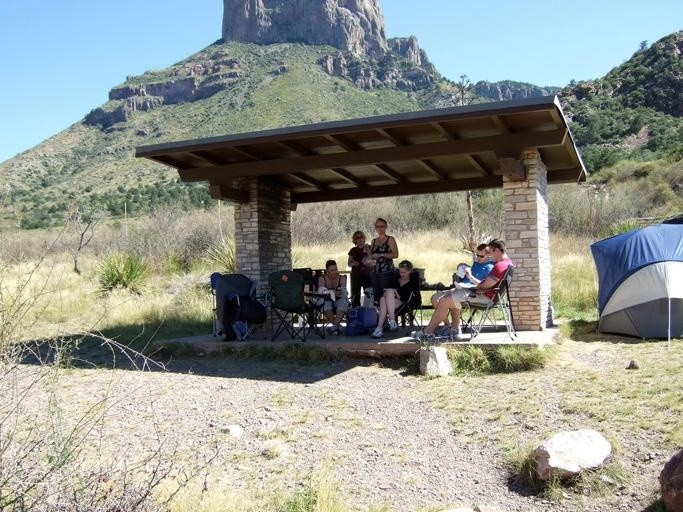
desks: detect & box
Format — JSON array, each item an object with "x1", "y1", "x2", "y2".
[{"x1": 395, "y1": 285, "x2": 453, "y2": 330}]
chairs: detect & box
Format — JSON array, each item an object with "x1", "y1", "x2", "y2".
[
  {"x1": 447, "y1": 264, "x2": 516, "y2": 343},
  {"x1": 210, "y1": 272, "x2": 270, "y2": 344},
  {"x1": 373, "y1": 269, "x2": 420, "y2": 336},
  {"x1": 268, "y1": 267, "x2": 348, "y2": 342}
]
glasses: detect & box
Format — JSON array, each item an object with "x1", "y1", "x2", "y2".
[
  {"x1": 399, "y1": 263, "x2": 410, "y2": 268},
  {"x1": 477, "y1": 254, "x2": 484, "y2": 258}
]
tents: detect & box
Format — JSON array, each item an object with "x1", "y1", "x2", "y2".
[{"x1": 586, "y1": 222, "x2": 683, "y2": 342}]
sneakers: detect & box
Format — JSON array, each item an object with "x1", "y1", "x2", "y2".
[
  {"x1": 389, "y1": 323, "x2": 399, "y2": 331},
  {"x1": 329, "y1": 325, "x2": 345, "y2": 336},
  {"x1": 411, "y1": 326, "x2": 462, "y2": 343},
  {"x1": 371, "y1": 328, "x2": 384, "y2": 338}
]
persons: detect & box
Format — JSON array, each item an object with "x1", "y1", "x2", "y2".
[
  {"x1": 370, "y1": 259, "x2": 421, "y2": 339},
  {"x1": 346, "y1": 229, "x2": 371, "y2": 306},
  {"x1": 362, "y1": 217, "x2": 398, "y2": 303},
  {"x1": 411, "y1": 238, "x2": 513, "y2": 340},
  {"x1": 430, "y1": 242, "x2": 493, "y2": 334},
  {"x1": 318, "y1": 259, "x2": 347, "y2": 335}
]
